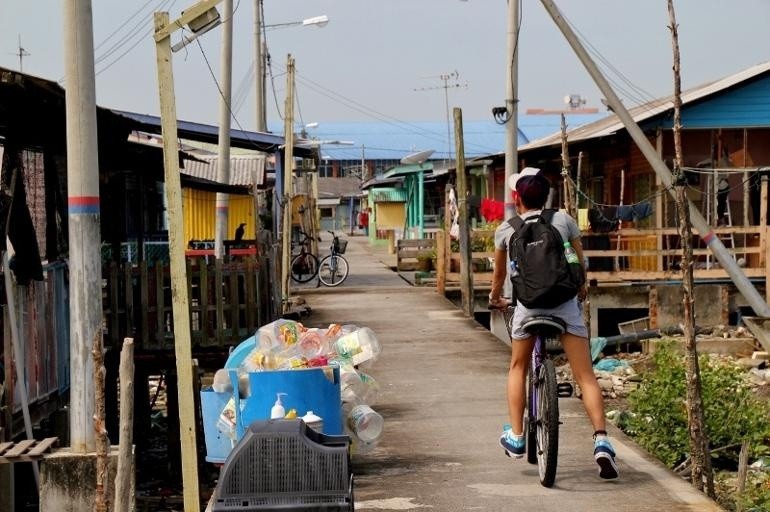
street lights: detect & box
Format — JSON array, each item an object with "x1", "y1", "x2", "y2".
[
  {"x1": 279, "y1": 140, "x2": 355, "y2": 312},
  {"x1": 254, "y1": 14, "x2": 331, "y2": 132},
  {"x1": 292, "y1": 122, "x2": 320, "y2": 137}
]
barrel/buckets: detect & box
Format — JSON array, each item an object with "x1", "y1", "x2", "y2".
[{"x1": 216, "y1": 318, "x2": 385, "y2": 443}]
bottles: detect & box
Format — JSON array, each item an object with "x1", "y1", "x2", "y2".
[
  {"x1": 285, "y1": 408, "x2": 297, "y2": 418},
  {"x1": 508, "y1": 261, "x2": 521, "y2": 278},
  {"x1": 563, "y1": 241, "x2": 580, "y2": 266},
  {"x1": 301, "y1": 411, "x2": 324, "y2": 433}
]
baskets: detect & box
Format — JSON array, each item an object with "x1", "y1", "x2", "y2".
[{"x1": 334, "y1": 239, "x2": 348, "y2": 254}]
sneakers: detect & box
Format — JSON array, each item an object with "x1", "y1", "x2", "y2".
[
  {"x1": 594, "y1": 440, "x2": 619, "y2": 481},
  {"x1": 499, "y1": 430, "x2": 526, "y2": 456}
]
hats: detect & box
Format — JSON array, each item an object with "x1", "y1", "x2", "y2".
[{"x1": 516, "y1": 175, "x2": 549, "y2": 196}]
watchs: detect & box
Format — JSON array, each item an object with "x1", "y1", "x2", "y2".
[{"x1": 488, "y1": 294, "x2": 501, "y2": 304}]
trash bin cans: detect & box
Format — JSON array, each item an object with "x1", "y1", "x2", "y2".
[{"x1": 210, "y1": 417, "x2": 354, "y2": 512}]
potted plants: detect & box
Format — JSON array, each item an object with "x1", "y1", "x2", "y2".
[{"x1": 416, "y1": 233, "x2": 487, "y2": 272}]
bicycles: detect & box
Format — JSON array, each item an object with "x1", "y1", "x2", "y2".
[
  {"x1": 290, "y1": 231, "x2": 320, "y2": 284},
  {"x1": 487, "y1": 291, "x2": 587, "y2": 487},
  {"x1": 318, "y1": 230, "x2": 350, "y2": 288}
]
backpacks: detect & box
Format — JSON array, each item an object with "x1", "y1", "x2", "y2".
[{"x1": 507, "y1": 209, "x2": 585, "y2": 309}]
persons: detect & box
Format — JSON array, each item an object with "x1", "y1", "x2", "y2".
[{"x1": 488, "y1": 174, "x2": 619, "y2": 479}]
